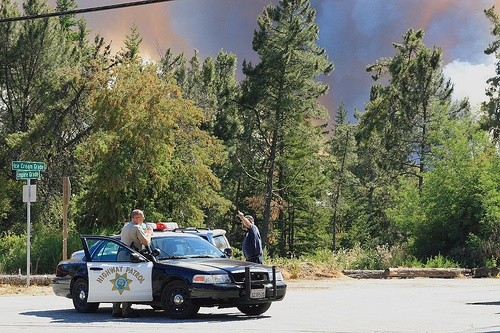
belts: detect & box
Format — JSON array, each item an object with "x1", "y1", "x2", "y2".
[{"x1": 246, "y1": 255, "x2": 260, "y2": 261}]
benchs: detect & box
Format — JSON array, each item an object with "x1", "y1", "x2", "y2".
[{"x1": 98, "y1": 254, "x2": 117, "y2": 261}]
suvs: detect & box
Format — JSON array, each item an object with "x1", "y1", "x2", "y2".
[{"x1": 70, "y1": 223, "x2": 233, "y2": 260}]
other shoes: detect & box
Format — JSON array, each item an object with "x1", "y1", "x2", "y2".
[
  {"x1": 112, "y1": 312, "x2": 123, "y2": 318},
  {"x1": 122, "y1": 310, "x2": 140, "y2": 318}
]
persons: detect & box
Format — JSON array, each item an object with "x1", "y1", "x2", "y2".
[
  {"x1": 236, "y1": 210, "x2": 263, "y2": 264},
  {"x1": 112, "y1": 209, "x2": 153, "y2": 318}
]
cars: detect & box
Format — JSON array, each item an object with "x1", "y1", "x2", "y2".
[{"x1": 51, "y1": 232, "x2": 287, "y2": 320}]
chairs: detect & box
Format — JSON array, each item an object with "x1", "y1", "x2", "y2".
[{"x1": 160, "y1": 239, "x2": 177, "y2": 257}]
